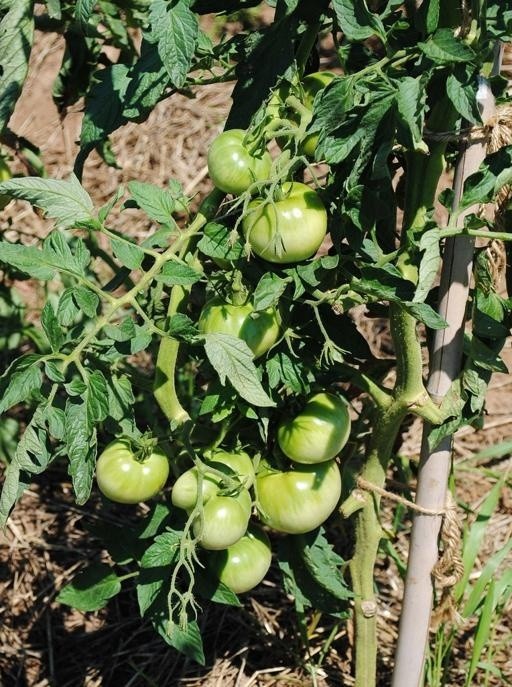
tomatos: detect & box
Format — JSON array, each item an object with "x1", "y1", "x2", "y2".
[{"x1": 95, "y1": 72, "x2": 352, "y2": 593}]
